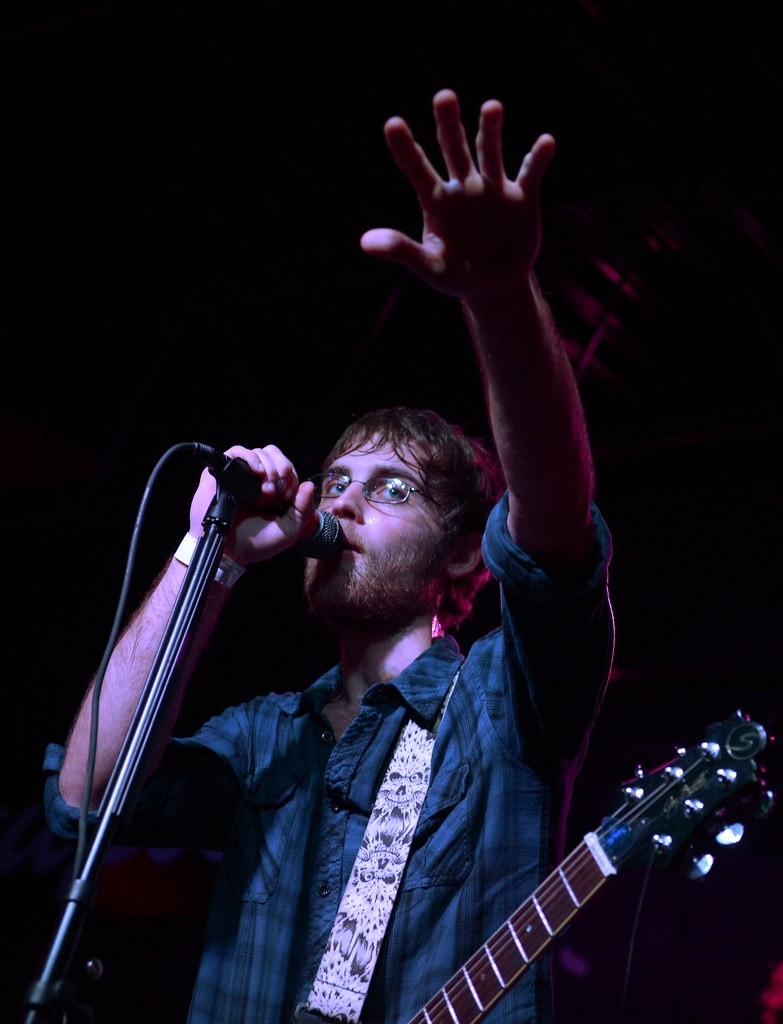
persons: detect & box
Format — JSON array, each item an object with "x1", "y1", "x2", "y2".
[{"x1": 41, "y1": 88, "x2": 614, "y2": 1024}]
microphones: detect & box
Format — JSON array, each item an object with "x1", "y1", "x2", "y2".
[{"x1": 191, "y1": 442, "x2": 343, "y2": 558}]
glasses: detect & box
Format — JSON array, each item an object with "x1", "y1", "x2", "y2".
[{"x1": 306, "y1": 472, "x2": 449, "y2": 515}]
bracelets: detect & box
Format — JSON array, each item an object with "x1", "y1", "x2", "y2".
[{"x1": 174, "y1": 532, "x2": 247, "y2": 587}]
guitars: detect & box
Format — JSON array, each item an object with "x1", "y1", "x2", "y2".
[{"x1": 400, "y1": 707, "x2": 773, "y2": 1022}]
